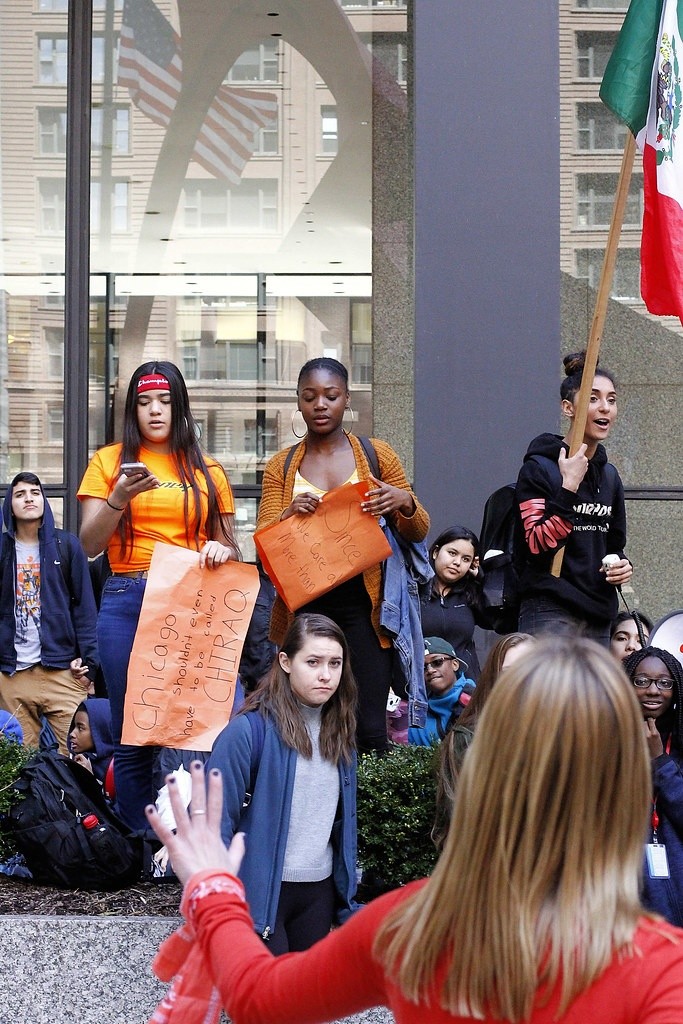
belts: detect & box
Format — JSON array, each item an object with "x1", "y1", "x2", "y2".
[{"x1": 112, "y1": 572, "x2": 149, "y2": 579}]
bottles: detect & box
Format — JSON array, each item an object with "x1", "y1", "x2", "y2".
[{"x1": 83, "y1": 815, "x2": 132, "y2": 875}]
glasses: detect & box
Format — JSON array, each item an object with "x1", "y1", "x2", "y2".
[
  {"x1": 632, "y1": 675, "x2": 676, "y2": 690},
  {"x1": 424, "y1": 657, "x2": 455, "y2": 672}
]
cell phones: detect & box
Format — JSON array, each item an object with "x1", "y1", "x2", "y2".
[{"x1": 121, "y1": 463, "x2": 160, "y2": 485}]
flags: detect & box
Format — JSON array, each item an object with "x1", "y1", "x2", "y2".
[{"x1": 599, "y1": 0, "x2": 683, "y2": 328}]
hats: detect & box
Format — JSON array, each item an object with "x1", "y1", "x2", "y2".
[{"x1": 421, "y1": 637, "x2": 469, "y2": 673}]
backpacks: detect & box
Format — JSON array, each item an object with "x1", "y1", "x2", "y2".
[
  {"x1": 9, "y1": 753, "x2": 144, "y2": 889},
  {"x1": 472, "y1": 455, "x2": 616, "y2": 636}
]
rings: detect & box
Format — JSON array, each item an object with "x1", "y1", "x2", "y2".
[{"x1": 191, "y1": 810, "x2": 206, "y2": 816}]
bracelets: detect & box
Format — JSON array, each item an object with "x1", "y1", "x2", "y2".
[{"x1": 107, "y1": 500, "x2": 125, "y2": 511}]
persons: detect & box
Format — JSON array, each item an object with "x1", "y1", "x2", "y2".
[
  {"x1": 610, "y1": 609, "x2": 654, "y2": 662},
  {"x1": 237, "y1": 557, "x2": 278, "y2": 699},
  {"x1": 408, "y1": 638, "x2": 476, "y2": 748},
  {"x1": 417, "y1": 525, "x2": 495, "y2": 687},
  {"x1": 255, "y1": 357, "x2": 430, "y2": 760},
  {"x1": 145, "y1": 638, "x2": 683, "y2": 1024},
  {"x1": 203, "y1": 614, "x2": 368, "y2": 958},
  {"x1": 621, "y1": 646, "x2": 683, "y2": 929},
  {"x1": 0, "y1": 472, "x2": 113, "y2": 764},
  {"x1": 66, "y1": 698, "x2": 116, "y2": 805},
  {"x1": 512, "y1": 350, "x2": 634, "y2": 650},
  {"x1": 76, "y1": 361, "x2": 243, "y2": 830}
]
